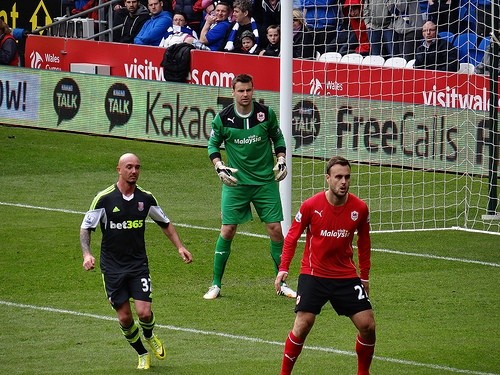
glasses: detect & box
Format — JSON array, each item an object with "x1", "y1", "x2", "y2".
[{"x1": 173, "y1": 19, "x2": 185, "y2": 22}]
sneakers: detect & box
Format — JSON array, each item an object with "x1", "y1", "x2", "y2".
[
  {"x1": 143, "y1": 333, "x2": 166, "y2": 359},
  {"x1": 277, "y1": 284, "x2": 297, "y2": 298},
  {"x1": 203, "y1": 285, "x2": 221, "y2": 300},
  {"x1": 137, "y1": 353, "x2": 150, "y2": 370}
]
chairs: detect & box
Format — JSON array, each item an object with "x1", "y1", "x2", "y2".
[{"x1": 188, "y1": 1, "x2": 495, "y2": 71}]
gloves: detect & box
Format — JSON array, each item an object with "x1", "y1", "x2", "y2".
[
  {"x1": 215, "y1": 160, "x2": 238, "y2": 188},
  {"x1": 272, "y1": 156, "x2": 287, "y2": 182}
]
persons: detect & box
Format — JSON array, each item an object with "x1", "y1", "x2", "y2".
[
  {"x1": 199, "y1": 74, "x2": 299, "y2": 299},
  {"x1": 78, "y1": 151, "x2": 195, "y2": 371},
  {"x1": 0, "y1": 19, "x2": 20, "y2": 66},
  {"x1": 68, "y1": 0, "x2": 499, "y2": 78},
  {"x1": 273, "y1": 157, "x2": 380, "y2": 375}
]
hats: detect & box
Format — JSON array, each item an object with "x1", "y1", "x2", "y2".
[{"x1": 241, "y1": 30, "x2": 255, "y2": 41}]
